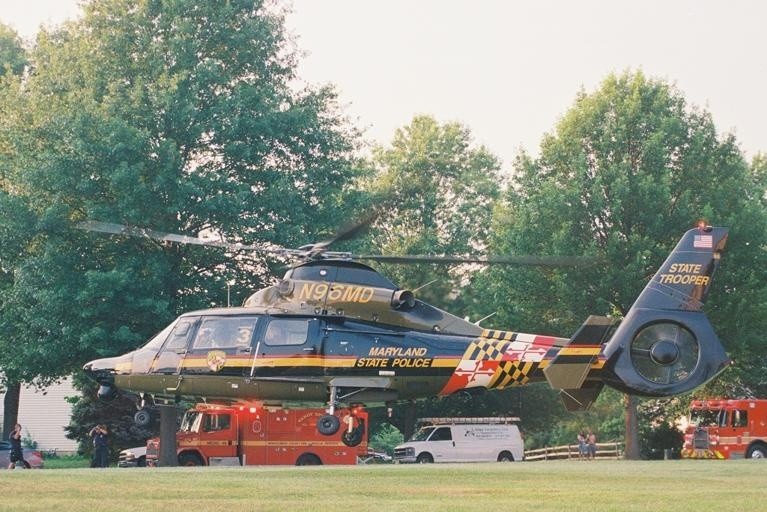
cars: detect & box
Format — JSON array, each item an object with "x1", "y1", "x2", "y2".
[
  {"x1": 0, "y1": 439, "x2": 45, "y2": 469},
  {"x1": 117, "y1": 446, "x2": 149, "y2": 468}
]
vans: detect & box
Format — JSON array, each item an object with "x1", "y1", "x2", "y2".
[{"x1": 391, "y1": 421, "x2": 526, "y2": 464}]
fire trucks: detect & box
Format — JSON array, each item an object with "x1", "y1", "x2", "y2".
[
  {"x1": 681, "y1": 398, "x2": 767, "y2": 459},
  {"x1": 143, "y1": 402, "x2": 368, "y2": 468}
]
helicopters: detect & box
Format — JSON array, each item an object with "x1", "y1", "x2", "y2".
[{"x1": 72, "y1": 206, "x2": 732, "y2": 446}]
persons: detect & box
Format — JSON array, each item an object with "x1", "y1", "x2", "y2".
[
  {"x1": 7, "y1": 424, "x2": 32, "y2": 470},
  {"x1": 577, "y1": 429, "x2": 598, "y2": 460},
  {"x1": 89, "y1": 424, "x2": 112, "y2": 468}
]
